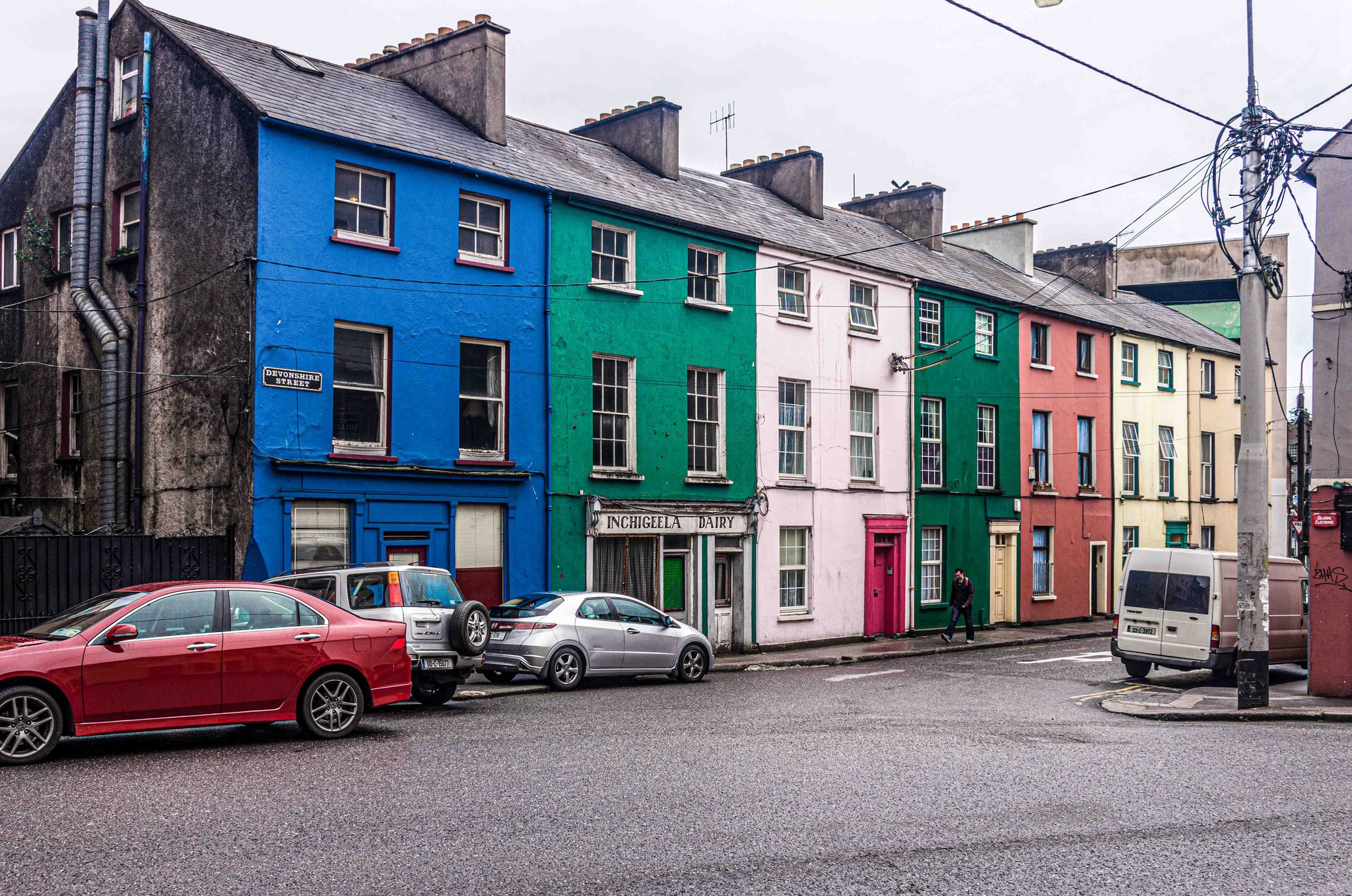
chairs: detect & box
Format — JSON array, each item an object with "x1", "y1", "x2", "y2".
[
  {"x1": 594, "y1": 602, "x2": 611, "y2": 620},
  {"x1": 173, "y1": 611, "x2": 205, "y2": 635},
  {"x1": 301, "y1": 588, "x2": 374, "y2": 608}
]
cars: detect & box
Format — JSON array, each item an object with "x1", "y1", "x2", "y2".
[
  {"x1": 0, "y1": 578, "x2": 414, "y2": 765},
  {"x1": 474, "y1": 590, "x2": 715, "y2": 691}
]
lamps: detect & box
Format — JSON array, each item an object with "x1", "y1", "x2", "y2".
[{"x1": 462, "y1": 400, "x2": 486, "y2": 450}]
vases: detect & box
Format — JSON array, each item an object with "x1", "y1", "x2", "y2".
[
  {"x1": 1079, "y1": 488, "x2": 1095, "y2": 494},
  {"x1": 1033, "y1": 485, "x2": 1052, "y2": 492},
  {"x1": 5, "y1": 473, "x2": 18, "y2": 481}
]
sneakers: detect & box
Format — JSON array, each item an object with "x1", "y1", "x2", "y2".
[
  {"x1": 966, "y1": 639, "x2": 975, "y2": 643},
  {"x1": 941, "y1": 633, "x2": 951, "y2": 643}
]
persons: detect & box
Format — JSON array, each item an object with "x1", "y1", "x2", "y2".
[{"x1": 941, "y1": 568, "x2": 975, "y2": 644}]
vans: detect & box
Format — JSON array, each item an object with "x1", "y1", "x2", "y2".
[{"x1": 1110, "y1": 546, "x2": 1309, "y2": 687}]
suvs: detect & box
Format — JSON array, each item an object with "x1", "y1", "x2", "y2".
[{"x1": 229, "y1": 561, "x2": 492, "y2": 704}]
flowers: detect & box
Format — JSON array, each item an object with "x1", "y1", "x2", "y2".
[
  {"x1": 7, "y1": 454, "x2": 17, "y2": 474},
  {"x1": 1033, "y1": 481, "x2": 1053, "y2": 487},
  {"x1": 1078, "y1": 483, "x2": 1096, "y2": 489}
]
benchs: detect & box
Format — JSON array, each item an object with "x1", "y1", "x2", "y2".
[{"x1": 248, "y1": 608, "x2": 312, "y2": 630}]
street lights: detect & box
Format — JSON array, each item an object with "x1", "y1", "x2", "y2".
[{"x1": 1032, "y1": 0, "x2": 1270, "y2": 709}]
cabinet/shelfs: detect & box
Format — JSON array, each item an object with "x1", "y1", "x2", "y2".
[{"x1": 334, "y1": 412, "x2": 362, "y2": 440}]
